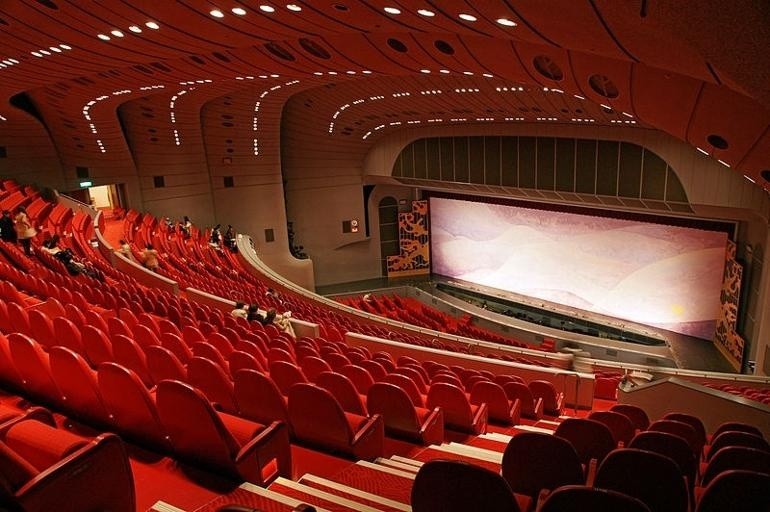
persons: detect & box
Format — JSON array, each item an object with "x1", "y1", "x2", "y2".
[
  {"x1": 363, "y1": 292, "x2": 373, "y2": 305},
  {"x1": 0, "y1": 206, "x2": 86, "y2": 276},
  {"x1": 116, "y1": 239, "x2": 142, "y2": 266},
  {"x1": 142, "y1": 243, "x2": 159, "y2": 272},
  {"x1": 266, "y1": 288, "x2": 279, "y2": 297},
  {"x1": 230, "y1": 300, "x2": 295, "y2": 341},
  {"x1": 165, "y1": 216, "x2": 238, "y2": 256}
]
